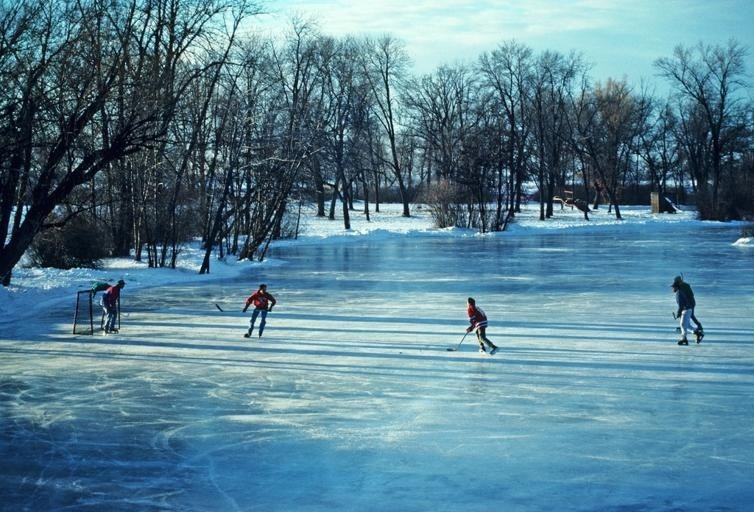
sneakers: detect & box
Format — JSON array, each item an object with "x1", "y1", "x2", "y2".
[{"x1": 104, "y1": 327, "x2": 119, "y2": 331}]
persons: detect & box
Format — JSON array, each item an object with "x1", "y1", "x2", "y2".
[
  {"x1": 100, "y1": 280, "x2": 125, "y2": 334},
  {"x1": 242, "y1": 284, "x2": 276, "y2": 338},
  {"x1": 466, "y1": 298, "x2": 497, "y2": 354},
  {"x1": 669, "y1": 281, "x2": 702, "y2": 343},
  {"x1": 675, "y1": 277, "x2": 703, "y2": 330}
]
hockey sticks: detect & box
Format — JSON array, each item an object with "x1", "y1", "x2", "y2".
[
  {"x1": 216, "y1": 304, "x2": 270, "y2": 312},
  {"x1": 672, "y1": 312, "x2": 680, "y2": 320},
  {"x1": 447, "y1": 332, "x2": 470, "y2": 351}
]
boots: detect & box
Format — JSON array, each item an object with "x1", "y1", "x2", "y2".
[
  {"x1": 479, "y1": 347, "x2": 499, "y2": 355},
  {"x1": 694, "y1": 327, "x2": 704, "y2": 343},
  {"x1": 678, "y1": 340, "x2": 688, "y2": 345}
]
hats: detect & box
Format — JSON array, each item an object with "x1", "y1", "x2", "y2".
[
  {"x1": 117, "y1": 280, "x2": 126, "y2": 284},
  {"x1": 669, "y1": 282, "x2": 679, "y2": 287}
]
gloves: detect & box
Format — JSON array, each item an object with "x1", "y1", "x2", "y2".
[{"x1": 677, "y1": 310, "x2": 680, "y2": 317}]
